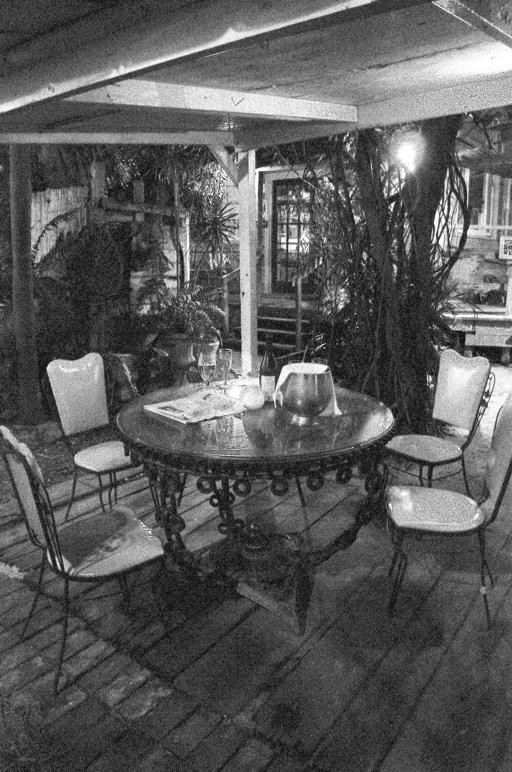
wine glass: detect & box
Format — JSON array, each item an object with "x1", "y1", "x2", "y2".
[
  {"x1": 197, "y1": 353, "x2": 217, "y2": 401},
  {"x1": 217, "y1": 348, "x2": 233, "y2": 389},
  {"x1": 280, "y1": 365, "x2": 334, "y2": 427}
]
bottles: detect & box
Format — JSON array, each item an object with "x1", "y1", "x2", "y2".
[{"x1": 258, "y1": 334, "x2": 277, "y2": 405}]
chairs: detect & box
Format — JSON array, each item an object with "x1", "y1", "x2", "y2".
[
  {"x1": 379, "y1": 347, "x2": 512, "y2": 633},
  {"x1": 45, "y1": 352, "x2": 148, "y2": 522},
  {"x1": 0, "y1": 425, "x2": 174, "y2": 698}
]
